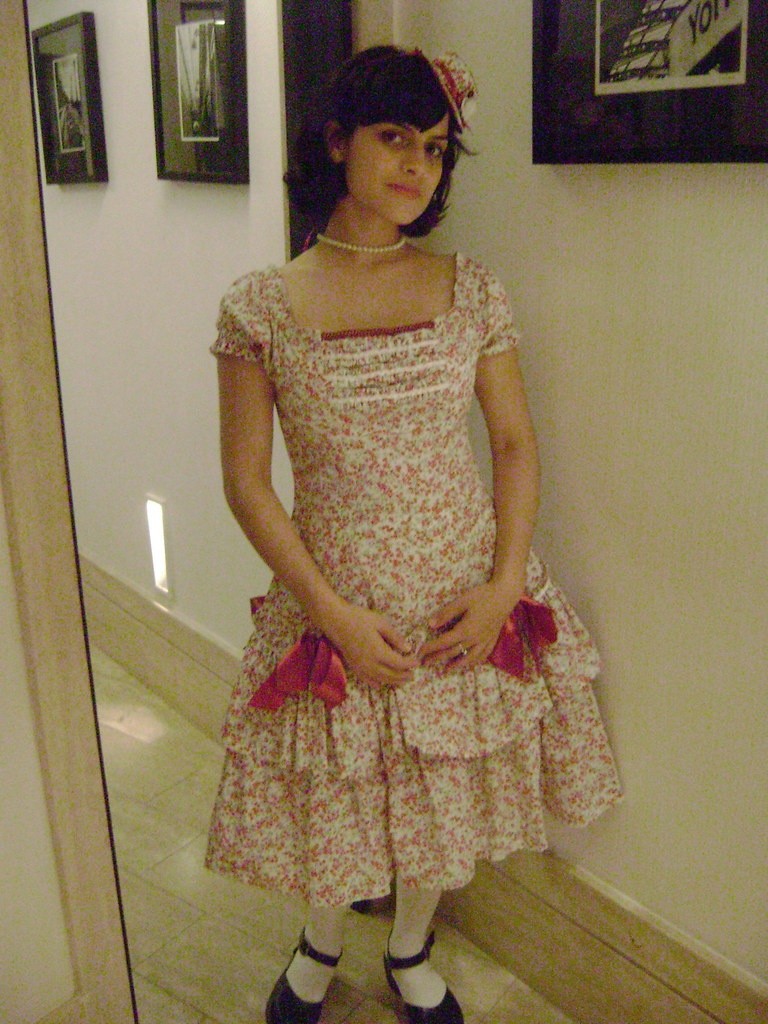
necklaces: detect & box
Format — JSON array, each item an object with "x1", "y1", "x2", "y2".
[{"x1": 318, "y1": 234, "x2": 407, "y2": 253}]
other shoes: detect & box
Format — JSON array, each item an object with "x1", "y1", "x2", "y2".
[
  {"x1": 382, "y1": 927, "x2": 465, "y2": 1024},
  {"x1": 265, "y1": 924, "x2": 344, "y2": 1024}
]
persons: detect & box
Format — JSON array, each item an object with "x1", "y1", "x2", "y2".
[{"x1": 205, "y1": 45, "x2": 625, "y2": 1024}]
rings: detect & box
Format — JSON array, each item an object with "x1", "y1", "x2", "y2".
[{"x1": 458, "y1": 643, "x2": 467, "y2": 656}]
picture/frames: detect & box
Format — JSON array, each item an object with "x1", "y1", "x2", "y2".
[
  {"x1": 532, "y1": 0, "x2": 768, "y2": 164},
  {"x1": 148, "y1": 0, "x2": 250, "y2": 186},
  {"x1": 32, "y1": 11, "x2": 107, "y2": 185}
]
